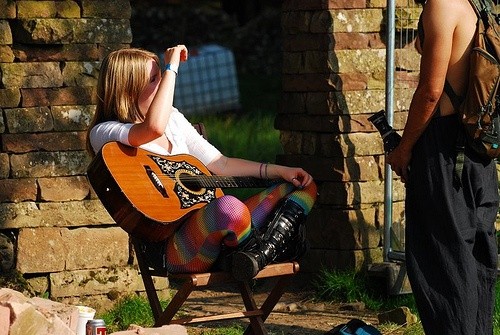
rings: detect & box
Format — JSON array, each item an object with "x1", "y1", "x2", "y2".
[{"x1": 291, "y1": 178, "x2": 296, "y2": 182}]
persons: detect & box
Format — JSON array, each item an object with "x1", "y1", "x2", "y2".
[
  {"x1": 386, "y1": 0, "x2": 500, "y2": 335},
  {"x1": 87, "y1": 45, "x2": 317, "y2": 279}
]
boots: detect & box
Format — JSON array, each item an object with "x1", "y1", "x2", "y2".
[{"x1": 229, "y1": 197, "x2": 306, "y2": 279}]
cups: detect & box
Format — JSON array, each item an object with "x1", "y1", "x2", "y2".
[{"x1": 74, "y1": 305, "x2": 96, "y2": 334}]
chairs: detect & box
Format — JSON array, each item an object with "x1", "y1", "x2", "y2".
[{"x1": 129, "y1": 236, "x2": 300, "y2": 335}]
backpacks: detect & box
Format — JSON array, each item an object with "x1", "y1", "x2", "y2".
[{"x1": 417, "y1": 0, "x2": 500, "y2": 159}]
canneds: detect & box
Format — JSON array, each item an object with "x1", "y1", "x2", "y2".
[{"x1": 86, "y1": 319, "x2": 107, "y2": 335}]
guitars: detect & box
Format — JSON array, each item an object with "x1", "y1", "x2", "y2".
[{"x1": 87, "y1": 141, "x2": 285, "y2": 246}]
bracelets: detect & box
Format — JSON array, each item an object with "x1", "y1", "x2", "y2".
[
  {"x1": 265, "y1": 163, "x2": 268, "y2": 180},
  {"x1": 260, "y1": 163, "x2": 263, "y2": 179},
  {"x1": 162, "y1": 64, "x2": 178, "y2": 76}
]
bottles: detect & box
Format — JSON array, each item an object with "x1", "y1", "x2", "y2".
[{"x1": 367, "y1": 109, "x2": 422, "y2": 183}]
